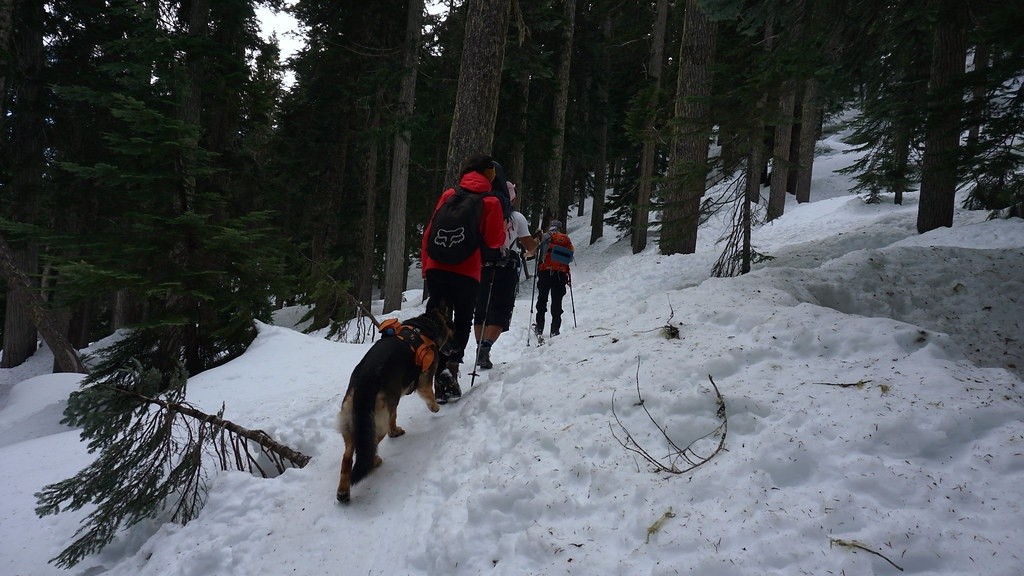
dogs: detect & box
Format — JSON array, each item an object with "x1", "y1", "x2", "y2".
[{"x1": 336, "y1": 308, "x2": 455, "y2": 503}]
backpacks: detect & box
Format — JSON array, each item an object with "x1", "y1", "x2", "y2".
[
  {"x1": 427, "y1": 186, "x2": 495, "y2": 264},
  {"x1": 545, "y1": 231, "x2": 574, "y2": 271}
]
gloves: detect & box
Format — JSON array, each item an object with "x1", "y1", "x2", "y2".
[{"x1": 534, "y1": 231, "x2": 543, "y2": 243}]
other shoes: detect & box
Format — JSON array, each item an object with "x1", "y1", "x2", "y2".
[
  {"x1": 549, "y1": 330, "x2": 560, "y2": 337},
  {"x1": 434, "y1": 381, "x2": 448, "y2": 405},
  {"x1": 437, "y1": 373, "x2": 462, "y2": 397},
  {"x1": 535, "y1": 328, "x2": 543, "y2": 336}
]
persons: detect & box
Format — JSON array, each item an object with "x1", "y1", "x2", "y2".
[
  {"x1": 421, "y1": 155, "x2": 507, "y2": 406},
  {"x1": 525, "y1": 219, "x2": 574, "y2": 338},
  {"x1": 474, "y1": 180, "x2": 543, "y2": 370}
]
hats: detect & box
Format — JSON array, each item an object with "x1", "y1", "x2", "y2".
[
  {"x1": 549, "y1": 220, "x2": 562, "y2": 228},
  {"x1": 505, "y1": 181, "x2": 516, "y2": 201}
]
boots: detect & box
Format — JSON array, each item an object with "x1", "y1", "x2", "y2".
[{"x1": 476, "y1": 342, "x2": 493, "y2": 369}]
